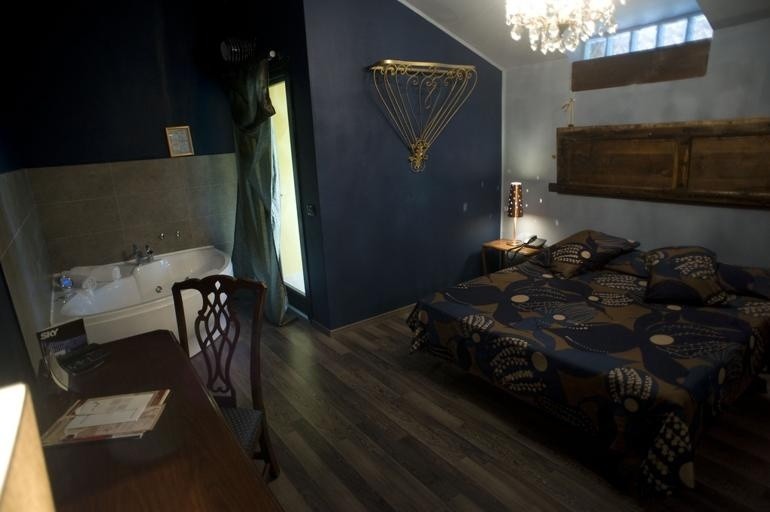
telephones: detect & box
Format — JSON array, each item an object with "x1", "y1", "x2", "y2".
[
  {"x1": 61, "y1": 343, "x2": 111, "y2": 372},
  {"x1": 524, "y1": 234, "x2": 547, "y2": 248}
]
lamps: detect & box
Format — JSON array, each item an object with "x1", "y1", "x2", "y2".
[
  {"x1": 507, "y1": 178, "x2": 522, "y2": 247},
  {"x1": 503, "y1": 0, "x2": 626, "y2": 56}
]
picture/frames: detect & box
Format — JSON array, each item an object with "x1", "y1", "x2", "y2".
[{"x1": 164, "y1": 125, "x2": 195, "y2": 158}]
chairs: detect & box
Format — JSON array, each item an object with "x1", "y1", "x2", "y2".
[{"x1": 170, "y1": 274, "x2": 280, "y2": 486}]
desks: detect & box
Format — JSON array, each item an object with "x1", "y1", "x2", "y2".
[
  {"x1": 483, "y1": 237, "x2": 542, "y2": 272},
  {"x1": 30, "y1": 326, "x2": 281, "y2": 512}
]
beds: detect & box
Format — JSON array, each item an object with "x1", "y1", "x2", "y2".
[{"x1": 405, "y1": 245, "x2": 770, "y2": 512}]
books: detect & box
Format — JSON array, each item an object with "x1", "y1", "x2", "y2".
[
  {"x1": 36, "y1": 316, "x2": 89, "y2": 372},
  {"x1": 42, "y1": 344, "x2": 174, "y2": 449}
]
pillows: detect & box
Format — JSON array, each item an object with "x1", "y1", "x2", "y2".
[
  {"x1": 526, "y1": 230, "x2": 641, "y2": 282},
  {"x1": 641, "y1": 244, "x2": 736, "y2": 305}
]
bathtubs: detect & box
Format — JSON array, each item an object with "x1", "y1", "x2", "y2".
[{"x1": 50, "y1": 245, "x2": 234, "y2": 358}]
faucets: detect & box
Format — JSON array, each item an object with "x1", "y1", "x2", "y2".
[
  {"x1": 145, "y1": 245, "x2": 154, "y2": 262},
  {"x1": 129, "y1": 243, "x2": 143, "y2": 264}
]
